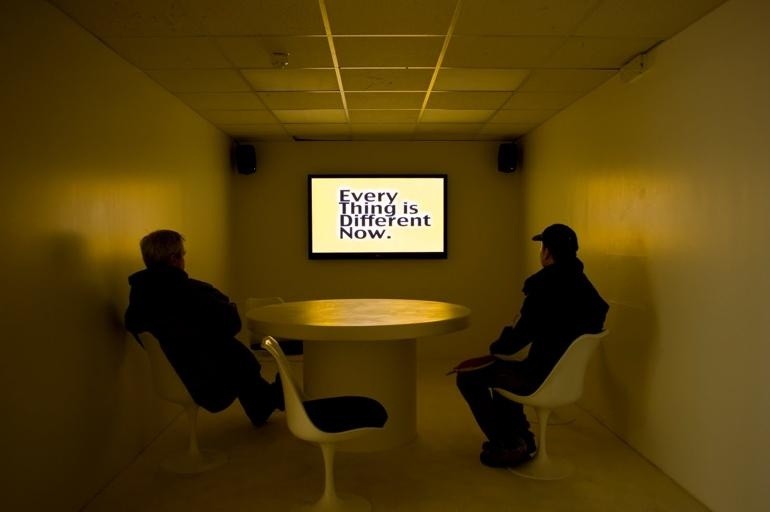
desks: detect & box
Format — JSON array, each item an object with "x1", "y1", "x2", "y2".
[{"x1": 247, "y1": 298, "x2": 471, "y2": 451}]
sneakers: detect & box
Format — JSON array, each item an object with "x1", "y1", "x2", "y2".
[{"x1": 480, "y1": 448, "x2": 525, "y2": 467}]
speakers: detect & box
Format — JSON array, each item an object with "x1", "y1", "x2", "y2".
[
  {"x1": 235, "y1": 144, "x2": 256, "y2": 175},
  {"x1": 497, "y1": 143, "x2": 519, "y2": 173}
]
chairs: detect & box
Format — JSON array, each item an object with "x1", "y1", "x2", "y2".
[
  {"x1": 496, "y1": 329, "x2": 611, "y2": 481},
  {"x1": 117, "y1": 332, "x2": 213, "y2": 479},
  {"x1": 248, "y1": 332, "x2": 303, "y2": 357},
  {"x1": 257, "y1": 337, "x2": 389, "y2": 507}
]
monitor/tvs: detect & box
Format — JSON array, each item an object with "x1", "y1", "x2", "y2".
[{"x1": 308, "y1": 173, "x2": 448, "y2": 260}]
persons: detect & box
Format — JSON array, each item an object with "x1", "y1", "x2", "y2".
[
  {"x1": 125, "y1": 229, "x2": 285, "y2": 428},
  {"x1": 456, "y1": 223, "x2": 609, "y2": 468}
]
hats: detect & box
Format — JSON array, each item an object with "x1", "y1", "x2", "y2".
[{"x1": 532, "y1": 223, "x2": 579, "y2": 250}]
